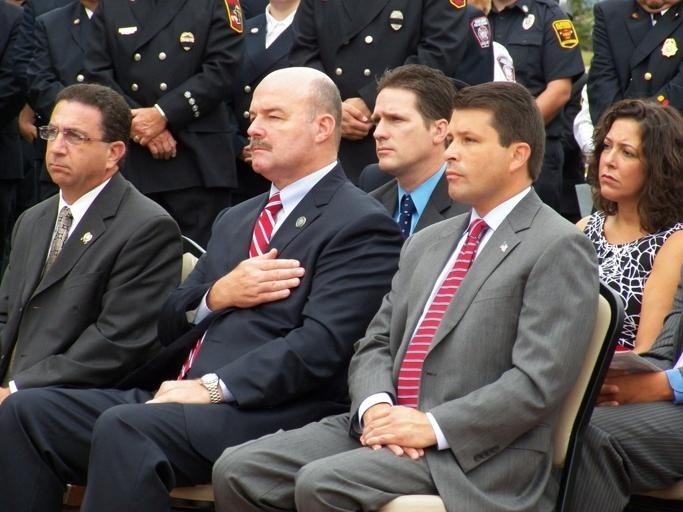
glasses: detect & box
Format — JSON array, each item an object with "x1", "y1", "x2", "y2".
[{"x1": 38, "y1": 124, "x2": 110, "y2": 147}]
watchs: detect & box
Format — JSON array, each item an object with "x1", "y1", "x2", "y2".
[{"x1": 198, "y1": 372, "x2": 219, "y2": 405}]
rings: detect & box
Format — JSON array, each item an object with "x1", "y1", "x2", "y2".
[{"x1": 135, "y1": 136, "x2": 139, "y2": 140}]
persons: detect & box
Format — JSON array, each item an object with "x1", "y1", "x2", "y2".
[
  {"x1": 572, "y1": 97, "x2": 682, "y2": 354},
  {"x1": 2, "y1": 4, "x2": 586, "y2": 273},
  {"x1": 562, "y1": 263, "x2": 683, "y2": 512},
  {"x1": 1, "y1": 66, "x2": 400, "y2": 511},
  {"x1": 587, "y1": 3, "x2": 680, "y2": 126},
  {"x1": 358, "y1": 63, "x2": 469, "y2": 238},
  {"x1": 0, "y1": 83, "x2": 183, "y2": 400},
  {"x1": 213, "y1": 79, "x2": 601, "y2": 512}
]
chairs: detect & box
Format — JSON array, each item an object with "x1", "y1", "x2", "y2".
[
  {"x1": 378, "y1": 276, "x2": 626, "y2": 511},
  {"x1": 174, "y1": 235, "x2": 210, "y2": 286}
]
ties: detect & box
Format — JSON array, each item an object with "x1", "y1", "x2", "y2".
[
  {"x1": 42, "y1": 205, "x2": 74, "y2": 283},
  {"x1": 398, "y1": 193, "x2": 418, "y2": 242},
  {"x1": 176, "y1": 190, "x2": 284, "y2": 383},
  {"x1": 395, "y1": 217, "x2": 492, "y2": 413}
]
